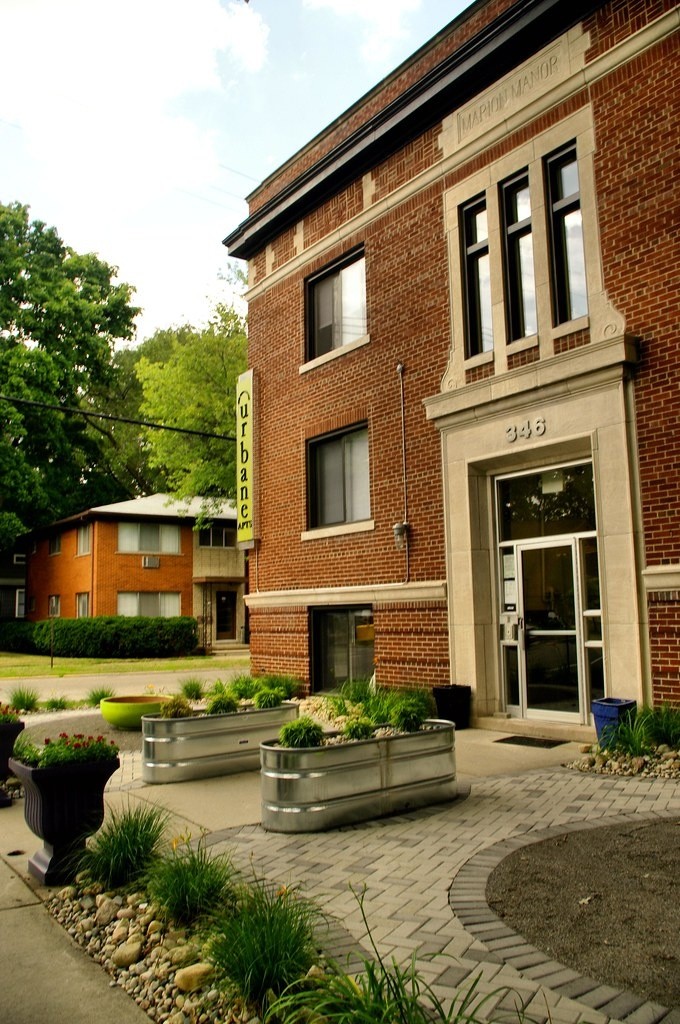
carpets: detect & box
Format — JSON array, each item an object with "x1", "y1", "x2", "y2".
[{"x1": 494, "y1": 736, "x2": 570, "y2": 749}]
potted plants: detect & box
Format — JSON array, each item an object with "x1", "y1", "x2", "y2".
[
  {"x1": 259, "y1": 700, "x2": 456, "y2": 832},
  {"x1": 141, "y1": 691, "x2": 300, "y2": 784}
]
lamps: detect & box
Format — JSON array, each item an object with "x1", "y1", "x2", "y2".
[{"x1": 392, "y1": 522, "x2": 405, "y2": 549}]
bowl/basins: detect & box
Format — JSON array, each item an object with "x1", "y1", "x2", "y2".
[{"x1": 100, "y1": 694, "x2": 175, "y2": 731}]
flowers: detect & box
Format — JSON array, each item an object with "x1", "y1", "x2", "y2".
[
  {"x1": 0, "y1": 702, "x2": 19, "y2": 724},
  {"x1": 24, "y1": 732, "x2": 118, "y2": 767}
]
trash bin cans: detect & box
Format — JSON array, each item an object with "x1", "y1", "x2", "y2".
[
  {"x1": 591, "y1": 697, "x2": 637, "y2": 751},
  {"x1": 433, "y1": 684, "x2": 471, "y2": 730}
]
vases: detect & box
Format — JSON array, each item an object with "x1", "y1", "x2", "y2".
[
  {"x1": 100, "y1": 695, "x2": 173, "y2": 731},
  {"x1": 9, "y1": 758, "x2": 120, "y2": 885},
  {"x1": 592, "y1": 698, "x2": 636, "y2": 750},
  {"x1": 433, "y1": 685, "x2": 471, "y2": 729},
  {"x1": 0, "y1": 723, "x2": 24, "y2": 807}
]
